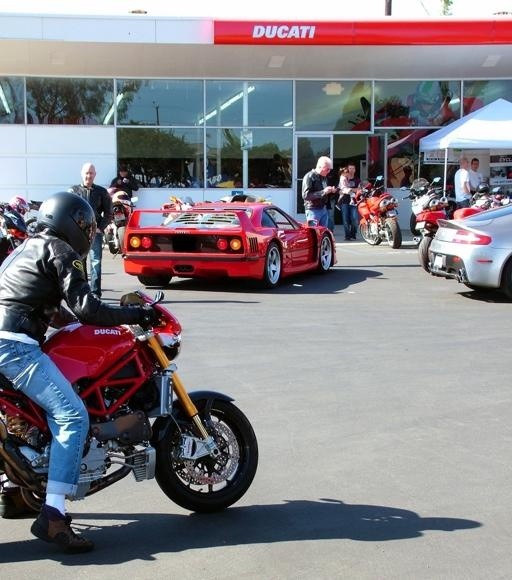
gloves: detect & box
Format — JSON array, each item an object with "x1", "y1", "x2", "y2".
[{"x1": 139, "y1": 305, "x2": 162, "y2": 329}]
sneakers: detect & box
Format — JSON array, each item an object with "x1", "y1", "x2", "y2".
[
  {"x1": 335, "y1": 204, "x2": 341, "y2": 211},
  {"x1": 349, "y1": 202, "x2": 358, "y2": 207},
  {"x1": 31, "y1": 503, "x2": 94, "y2": 554},
  {"x1": 344, "y1": 236, "x2": 356, "y2": 241}
]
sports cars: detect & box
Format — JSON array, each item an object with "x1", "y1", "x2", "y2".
[{"x1": 112, "y1": 197, "x2": 341, "y2": 284}]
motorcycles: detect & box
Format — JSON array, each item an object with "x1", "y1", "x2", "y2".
[
  {"x1": 1, "y1": 288, "x2": 259, "y2": 521},
  {"x1": 0, "y1": 196, "x2": 139, "y2": 278},
  {"x1": 350, "y1": 176, "x2": 512, "y2": 272}
]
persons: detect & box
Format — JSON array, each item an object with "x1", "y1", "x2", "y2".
[
  {"x1": 392, "y1": 80, "x2": 458, "y2": 135},
  {"x1": 301, "y1": 156, "x2": 339, "y2": 232},
  {"x1": 468, "y1": 158, "x2": 483, "y2": 194},
  {"x1": 335, "y1": 166, "x2": 357, "y2": 210},
  {"x1": 108, "y1": 164, "x2": 139, "y2": 197},
  {"x1": 341, "y1": 163, "x2": 362, "y2": 240},
  {"x1": 67, "y1": 162, "x2": 112, "y2": 300},
  {"x1": 455, "y1": 158, "x2": 472, "y2": 206},
  {"x1": 0, "y1": 189, "x2": 156, "y2": 557},
  {"x1": 398, "y1": 165, "x2": 414, "y2": 187},
  {"x1": 0, "y1": 194, "x2": 30, "y2": 259}
]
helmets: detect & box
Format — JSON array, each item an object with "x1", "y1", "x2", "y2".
[
  {"x1": 492, "y1": 186, "x2": 505, "y2": 194},
  {"x1": 36, "y1": 191, "x2": 98, "y2": 260},
  {"x1": 112, "y1": 191, "x2": 130, "y2": 208},
  {"x1": 9, "y1": 197, "x2": 30, "y2": 218},
  {"x1": 477, "y1": 182, "x2": 490, "y2": 193}
]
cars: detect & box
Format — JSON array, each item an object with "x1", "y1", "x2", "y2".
[{"x1": 425, "y1": 200, "x2": 512, "y2": 303}]
